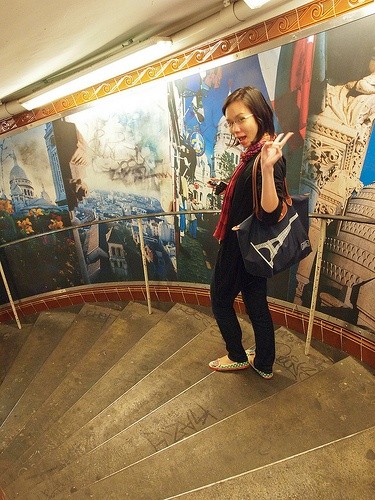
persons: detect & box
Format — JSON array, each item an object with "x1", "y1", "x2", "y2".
[{"x1": 208, "y1": 86, "x2": 308, "y2": 379}]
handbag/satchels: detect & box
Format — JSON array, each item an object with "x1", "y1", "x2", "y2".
[{"x1": 233, "y1": 147, "x2": 312, "y2": 277}]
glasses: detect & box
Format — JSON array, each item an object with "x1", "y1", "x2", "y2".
[{"x1": 223, "y1": 113, "x2": 258, "y2": 129}]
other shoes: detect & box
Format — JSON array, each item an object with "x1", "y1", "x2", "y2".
[
  {"x1": 244, "y1": 347, "x2": 274, "y2": 380},
  {"x1": 208, "y1": 352, "x2": 250, "y2": 373}
]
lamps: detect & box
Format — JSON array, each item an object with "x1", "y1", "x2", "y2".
[{"x1": 20, "y1": 36, "x2": 172, "y2": 111}]
